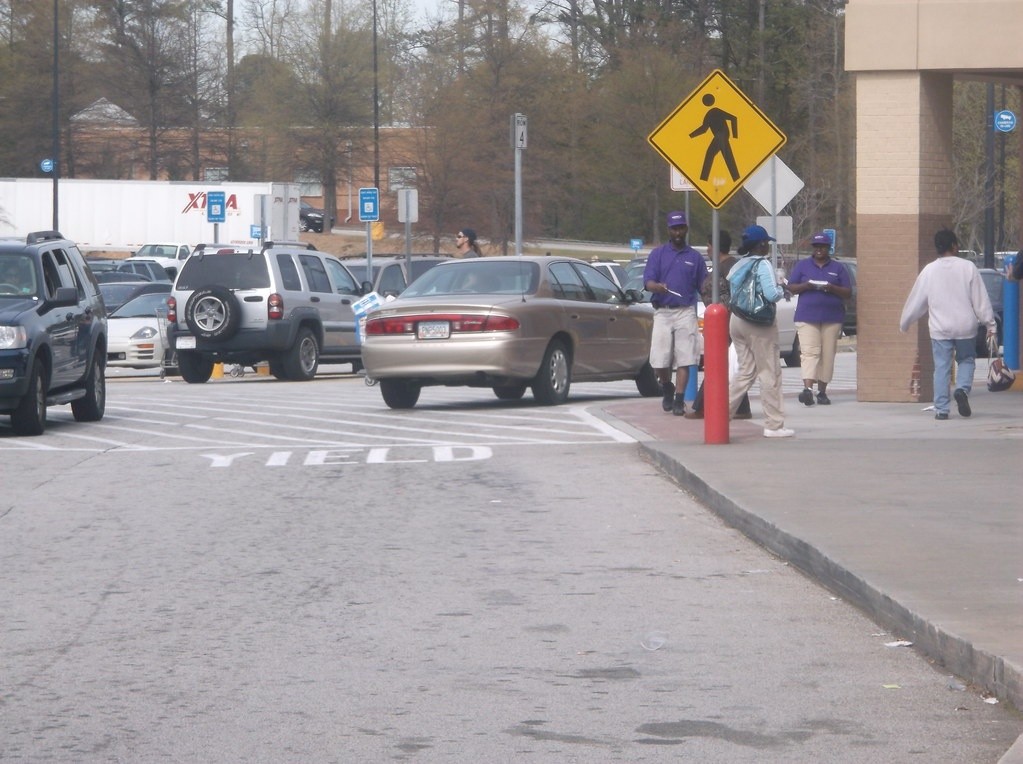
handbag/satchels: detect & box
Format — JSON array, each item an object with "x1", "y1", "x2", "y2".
[
  {"x1": 730, "y1": 257, "x2": 776, "y2": 326},
  {"x1": 987, "y1": 335, "x2": 1015, "y2": 392}
]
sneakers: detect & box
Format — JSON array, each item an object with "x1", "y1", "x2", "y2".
[
  {"x1": 662, "y1": 383, "x2": 676, "y2": 411},
  {"x1": 672, "y1": 399, "x2": 688, "y2": 416},
  {"x1": 763, "y1": 426, "x2": 795, "y2": 438},
  {"x1": 817, "y1": 392, "x2": 830, "y2": 405},
  {"x1": 686, "y1": 409, "x2": 704, "y2": 419},
  {"x1": 732, "y1": 411, "x2": 752, "y2": 419},
  {"x1": 799, "y1": 388, "x2": 815, "y2": 406}
]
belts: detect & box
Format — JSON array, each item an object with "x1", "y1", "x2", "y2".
[{"x1": 657, "y1": 305, "x2": 680, "y2": 309}]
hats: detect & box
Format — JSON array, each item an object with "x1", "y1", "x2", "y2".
[
  {"x1": 667, "y1": 212, "x2": 687, "y2": 227},
  {"x1": 741, "y1": 226, "x2": 776, "y2": 244},
  {"x1": 811, "y1": 232, "x2": 831, "y2": 245},
  {"x1": 463, "y1": 229, "x2": 476, "y2": 243}
]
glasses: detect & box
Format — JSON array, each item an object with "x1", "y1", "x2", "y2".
[{"x1": 457, "y1": 235, "x2": 466, "y2": 239}]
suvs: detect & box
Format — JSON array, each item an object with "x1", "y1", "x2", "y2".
[
  {"x1": 165, "y1": 237, "x2": 373, "y2": 386},
  {"x1": 0, "y1": 227, "x2": 109, "y2": 437},
  {"x1": 336, "y1": 249, "x2": 462, "y2": 315}
]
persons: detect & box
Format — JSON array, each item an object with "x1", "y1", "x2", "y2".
[
  {"x1": 1000, "y1": 248, "x2": 1023, "y2": 281},
  {"x1": 455, "y1": 228, "x2": 487, "y2": 292},
  {"x1": 728, "y1": 226, "x2": 794, "y2": 437},
  {"x1": 900, "y1": 229, "x2": 998, "y2": 419},
  {"x1": 786, "y1": 234, "x2": 852, "y2": 406},
  {"x1": 643, "y1": 211, "x2": 709, "y2": 415},
  {"x1": 0, "y1": 263, "x2": 32, "y2": 294},
  {"x1": 684, "y1": 229, "x2": 753, "y2": 419}
]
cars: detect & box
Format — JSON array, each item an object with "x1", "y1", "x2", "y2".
[
  {"x1": 299, "y1": 199, "x2": 335, "y2": 234},
  {"x1": 972, "y1": 267, "x2": 1007, "y2": 358},
  {"x1": 830, "y1": 256, "x2": 857, "y2": 338},
  {"x1": 358, "y1": 252, "x2": 669, "y2": 411},
  {"x1": 79, "y1": 240, "x2": 199, "y2": 378},
  {"x1": 580, "y1": 252, "x2": 802, "y2": 376}
]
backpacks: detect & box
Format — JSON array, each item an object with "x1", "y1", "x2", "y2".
[{"x1": 700, "y1": 273, "x2": 732, "y2": 312}]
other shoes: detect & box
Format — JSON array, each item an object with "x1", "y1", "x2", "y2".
[
  {"x1": 954, "y1": 389, "x2": 971, "y2": 416},
  {"x1": 936, "y1": 413, "x2": 948, "y2": 420}
]
